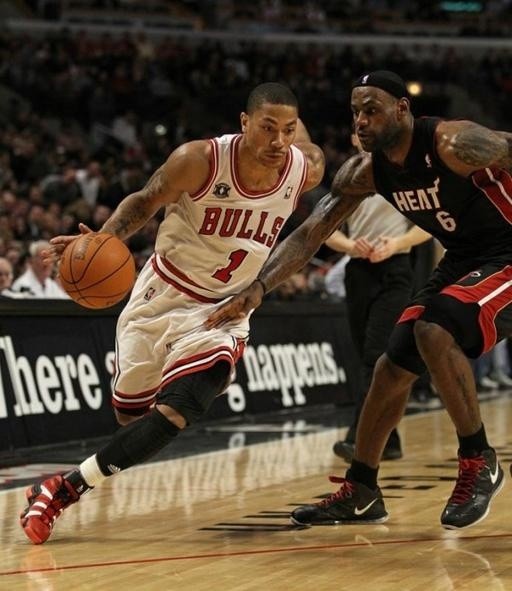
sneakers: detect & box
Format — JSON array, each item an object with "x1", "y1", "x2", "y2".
[
  {"x1": 21, "y1": 469, "x2": 95, "y2": 544},
  {"x1": 441, "y1": 447, "x2": 505, "y2": 529},
  {"x1": 290, "y1": 477, "x2": 389, "y2": 525},
  {"x1": 334, "y1": 440, "x2": 402, "y2": 463}
]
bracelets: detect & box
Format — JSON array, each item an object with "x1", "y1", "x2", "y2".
[{"x1": 254, "y1": 274, "x2": 270, "y2": 304}]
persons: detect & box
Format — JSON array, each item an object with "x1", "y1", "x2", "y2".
[
  {"x1": 202, "y1": 70, "x2": 512, "y2": 528},
  {"x1": 323, "y1": 121, "x2": 435, "y2": 461},
  {"x1": 19, "y1": 82, "x2": 327, "y2": 546}
]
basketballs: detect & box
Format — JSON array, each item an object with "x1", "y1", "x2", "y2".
[{"x1": 57, "y1": 232, "x2": 139, "y2": 310}]
